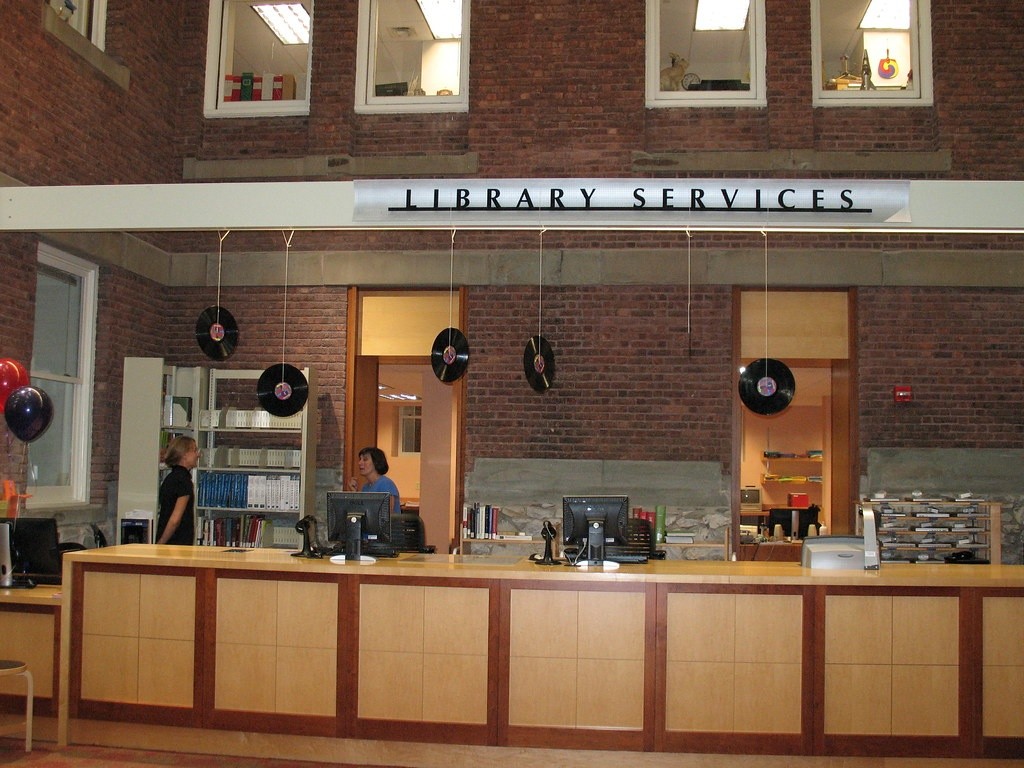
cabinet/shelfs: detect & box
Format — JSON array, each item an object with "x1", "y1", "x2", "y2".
[
  {"x1": 762, "y1": 449, "x2": 824, "y2": 508},
  {"x1": 651, "y1": 530, "x2": 731, "y2": 560},
  {"x1": 860, "y1": 498, "x2": 1004, "y2": 564},
  {"x1": 458, "y1": 521, "x2": 561, "y2": 561},
  {"x1": 115, "y1": 358, "x2": 319, "y2": 548}
]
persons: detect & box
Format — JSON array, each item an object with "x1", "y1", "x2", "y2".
[
  {"x1": 152, "y1": 434, "x2": 201, "y2": 547},
  {"x1": 348, "y1": 447, "x2": 402, "y2": 515}
]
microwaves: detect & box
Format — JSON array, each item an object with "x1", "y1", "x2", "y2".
[{"x1": 740, "y1": 486, "x2": 762, "y2": 511}]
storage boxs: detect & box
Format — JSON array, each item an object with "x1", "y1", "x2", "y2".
[{"x1": 788, "y1": 492, "x2": 810, "y2": 508}]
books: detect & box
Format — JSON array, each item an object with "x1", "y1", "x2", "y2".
[
  {"x1": 461, "y1": 501, "x2": 500, "y2": 541},
  {"x1": 665, "y1": 536, "x2": 695, "y2": 544},
  {"x1": 645, "y1": 512, "x2": 656, "y2": 532},
  {"x1": 495, "y1": 535, "x2": 533, "y2": 540},
  {"x1": 654, "y1": 504, "x2": 667, "y2": 544},
  {"x1": 632, "y1": 507, "x2": 642, "y2": 520},
  {"x1": 639, "y1": 511, "x2": 647, "y2": 521},
  {"x1": 496, "y1": 531, "x2": 526, "y2": 536},
  {"x1": 665, "y1": 530, "x2": 696, "y2": 537},
  {"x1": 195, "y1": 514, "x2": 273, "y2": 548},
  {"x1": 196, "y1": 470, "x2": 300, "y2": 511}
]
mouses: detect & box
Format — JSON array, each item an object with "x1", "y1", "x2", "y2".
[{"x1": 529, "y1": 553, "x2": 544, "y2": 560}]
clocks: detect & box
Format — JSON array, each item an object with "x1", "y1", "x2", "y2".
[{"x1": 682, "y1": 73, "x2": 701, "y2": 91}]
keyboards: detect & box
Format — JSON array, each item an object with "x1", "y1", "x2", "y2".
[
  {"x1": 329, "y1": 547, "x2": 400, "y2": 558},
  {"x1": 14, "y1": 575, "x2": 61, "y2": 585},
  {"x1": 580, "y1": 552, "x2": 649, "y2": 564}
]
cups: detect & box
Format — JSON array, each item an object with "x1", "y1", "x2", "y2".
[
  {"x1": 808, "y1": 524, "x2": 817, "y2": 536},
  {"x1": 773, "y1": 523, "x2": 783, "y2": 541}
]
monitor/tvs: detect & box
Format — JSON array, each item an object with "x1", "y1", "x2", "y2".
[
  {"x1": 0, "y1": 517, "x2": 61, "y2": 574},
  {"x1": 326, "y1": 491, "x2": 391, "y2": 542},
  {"x1": 563, "y1": 494, "x2": 629, "y2": 547}
]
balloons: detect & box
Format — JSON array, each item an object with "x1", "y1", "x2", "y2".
[
  {"x1": 3, "y1": 385, "x2": 54, "y2": 444},
  {"x1": 0, "y1": 358, "x2": 32, "y2": 414}
]
